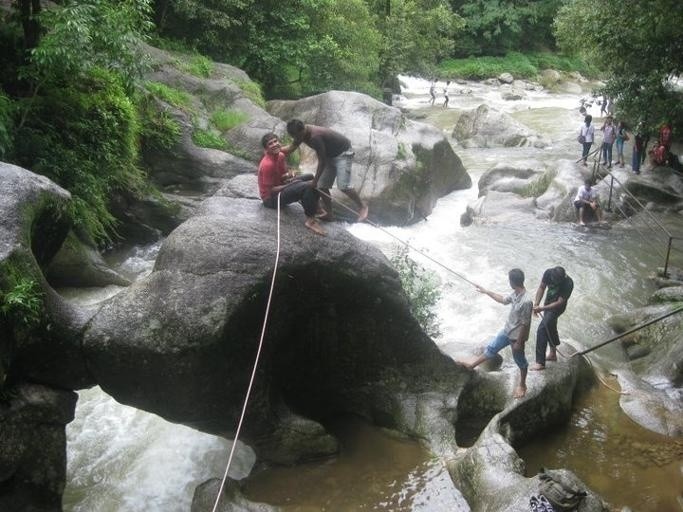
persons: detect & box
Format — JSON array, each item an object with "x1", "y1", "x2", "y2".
[
  {"x1": 578, "y1": 91, "x2": 671, "y2": 175},
  {"x1": 528, "y1": 265, "x2": 574, "y2": 371},
  {"x1": 256, "y1": 131, "x2": 327, "y2": 236},
  {"x1": 441, "y1": 80, "x2": 451, "y2": 110},
  {"x1": 573, "y1": 179, "x2": 609, "y2": 227},
  {"x1": 263, "y1": 118, "x2": 370, "y2": 222},
  {"x1": 427, "y1": 78, "x2": 438, "y2": 106},
  {"x1": 460, "y1": 268, "x2": 534, "y2": 397}
]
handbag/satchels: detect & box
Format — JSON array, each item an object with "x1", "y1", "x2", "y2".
[
  {"x1": 622, "y1": 132, "x2": 630, "y2": 140},
  {"x1": 577, "y1": 134, "x2": 587, "y2": 144}
]
